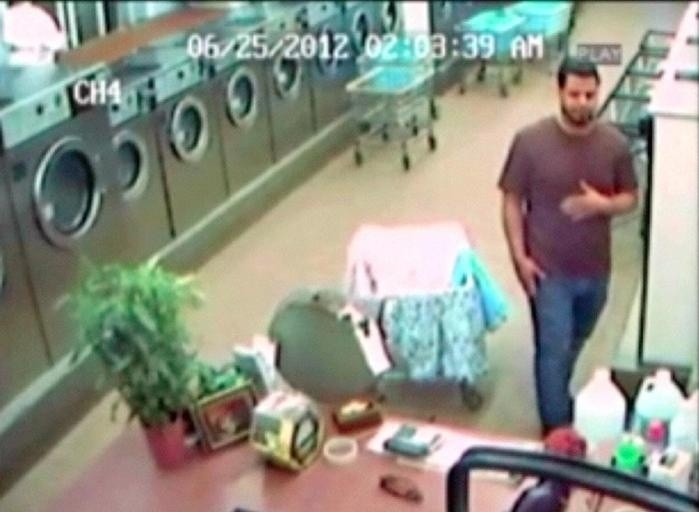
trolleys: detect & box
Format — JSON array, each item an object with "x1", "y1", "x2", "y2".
[{"x1": 347, "y1": 61, "x2": 436, "y2": 169}]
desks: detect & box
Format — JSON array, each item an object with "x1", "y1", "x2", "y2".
[{"x1": 41, "y1": 389, "x2": 655, "y2": 512}]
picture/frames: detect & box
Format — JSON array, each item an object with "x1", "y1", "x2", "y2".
[{"x1": 191, "y1": 382, "x2": 260, "y2": 456}]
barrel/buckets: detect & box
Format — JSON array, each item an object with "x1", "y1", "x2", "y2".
[
  {"x1": 629, "y1": 366, "x2": 690, "y2": 444},
  {"x1": 572, "y1": 366, "x2": 629, "y2": 447}
]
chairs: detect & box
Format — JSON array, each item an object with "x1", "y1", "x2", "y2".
[{"x1": 268, "y1": 287, "x2": 389, "y2": 412}]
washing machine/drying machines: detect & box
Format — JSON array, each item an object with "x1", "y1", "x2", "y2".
[
  {"x1": 105, "y1": 65, "x2": 175, "y2": 269},
  {"x1": 0, "y1": 58, "x2": 140, "y2": 368},
  {"x1": 188, "y1": 0, "x2": 268, "y2": 196},
  {"x1": 379, "y1": 1, "x2": 403, "y2": 39},
  {"x1": 127, "y1": 36, "x2": 229, "y2": 239},
  {"x1": 1, "y1": 161, "x2": 54, "y2": 409},
  {"x1": 342, "y1": 0, "x2": 385, "y2": 116},
  {"x1": 266, "y1": 1, "x2": 316, "y2": 175},
  {"x1": 304, "y1": 1, "x2": 359, "y2": 133}
]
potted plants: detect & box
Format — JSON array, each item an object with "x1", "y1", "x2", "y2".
[{"x1": 53, "y1": 249, "x2": 205, "y2": 472}]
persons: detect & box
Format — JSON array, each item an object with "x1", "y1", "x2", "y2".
[
  {"x1": 4, "y1": 0, "x2": 62, "y2": 69},
  {"x1": 498, "y1": 59, "x2": 638, "y2": 428}
]
